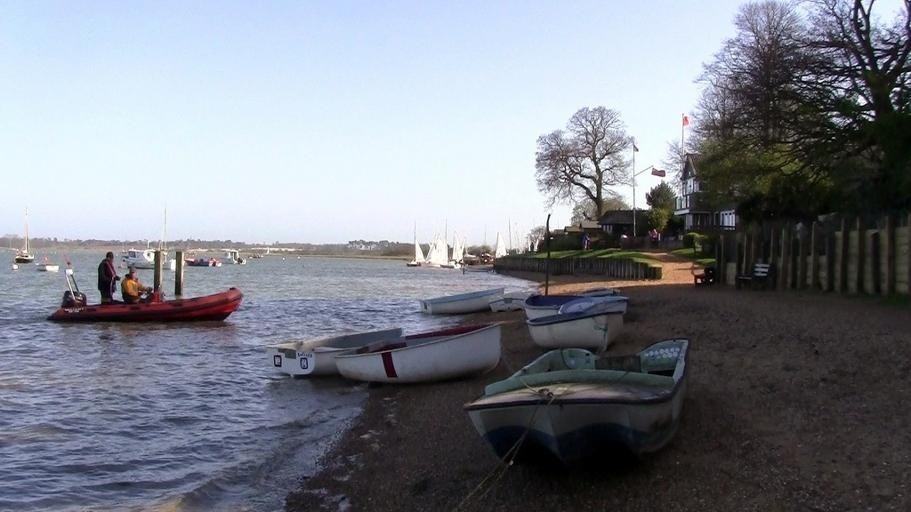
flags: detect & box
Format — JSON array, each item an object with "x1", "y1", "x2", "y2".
[
  {"x1": 651, "y1": 167, "x2": 665, "y2": 176},
  {"x1": 683, "y1": 116, "x2": 690, "y2": 126}
]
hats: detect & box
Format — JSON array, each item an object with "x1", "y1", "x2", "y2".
[{"x1": 129, "y1": 267, "x2": 137, "y2": 273}]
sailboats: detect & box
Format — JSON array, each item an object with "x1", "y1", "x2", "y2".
[
  {"x1": 15, "y1": 207, "x2": 34, "y2": 263},
  {"x1": 408, "y1": 218, "x2": 526, "y2": 271}
]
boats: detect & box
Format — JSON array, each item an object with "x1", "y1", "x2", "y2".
[
  {"x1": 461, "y1": 338, "x2": 690, "y2": 476},
  {"x1": 334, "y1": 320, "x2": 501, "y2": 384},
  {"x1": 526, "y1": 292, "x2": 629, "y2": 353},
  {"x1": 264, "y1": 326, "x2": 403, "y2": 379},
  {"x1": 38, "y1": 248, "x2": 243, "y2": 323},
  {"x1": 419, "y1": 287, "x2": 524, "y2": 313}
]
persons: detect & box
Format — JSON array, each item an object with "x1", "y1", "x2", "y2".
[
  {"x1": 120, "y1": 264, "x2": 147, "y2": 304},
  {"x1": 648, "y1": 227, "x2": 661, "y2": 248},
  {"x1": 582, "y1": 233, "x2": 592, "y2": 250},
  {"x1": 97, "y1": 252, "x2": 118, "y2": 302}
]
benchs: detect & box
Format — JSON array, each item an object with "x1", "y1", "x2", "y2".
[{"x1": 735, "y1": 263, "x2": 771, "y2": 289}]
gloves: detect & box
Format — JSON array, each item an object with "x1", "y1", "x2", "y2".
[{"x1": 116, "y1": 275, "x2": 120, "y2": 280}]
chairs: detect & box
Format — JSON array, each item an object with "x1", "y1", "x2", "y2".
[{"x1": 692, "y1": 265, "x2": 715, "y2": 287}]
